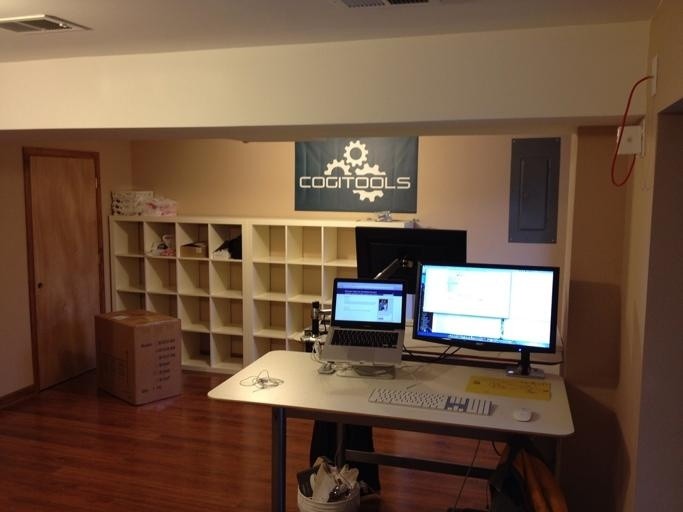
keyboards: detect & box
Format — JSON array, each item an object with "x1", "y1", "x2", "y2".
[{"x1": 368, "y1": 387, "x2": 492, "y2": 415}]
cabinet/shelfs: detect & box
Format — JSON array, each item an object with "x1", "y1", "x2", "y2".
[{"x1": 109, "y1": 215, "x2": 415, "y2": 376}]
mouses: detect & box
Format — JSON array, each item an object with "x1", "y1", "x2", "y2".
[{"x1": 514, "y1": 407, "x2": 531, "y2": 420}]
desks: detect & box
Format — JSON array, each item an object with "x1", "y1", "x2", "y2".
[
  {"x1": 286, "y1": 313, "x2": 564, "y2": 366},
  {"x1": 207, "y1": 350, "x2": 575, "y2": 510}
]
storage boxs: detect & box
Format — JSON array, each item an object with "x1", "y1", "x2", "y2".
[{"x1": 94, "y1": 307, "x2": 184, "y2": 406}]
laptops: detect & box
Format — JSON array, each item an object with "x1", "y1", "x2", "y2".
[{"x1": 319, "y1": 278, "x2": 406, "y2": 363}]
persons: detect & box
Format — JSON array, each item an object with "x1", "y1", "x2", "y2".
[
  {"x1": 378, "y1": 299, "x2": 383, "y2": 310},
  {"x1": 382, "y1": 300, "x2": 387, "y2": 311}
]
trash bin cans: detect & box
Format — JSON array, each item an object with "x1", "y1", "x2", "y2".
[{"x1": 298, "y1": 463, "x2": 361, "y2": 512}]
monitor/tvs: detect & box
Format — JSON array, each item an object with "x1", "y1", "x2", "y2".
[
  {"x1": 355, "y1": 227, "x2": 467, "y2": 326},
  {"x1": 412, "y1": 259, "x2": 560, "y2": 354}
]
